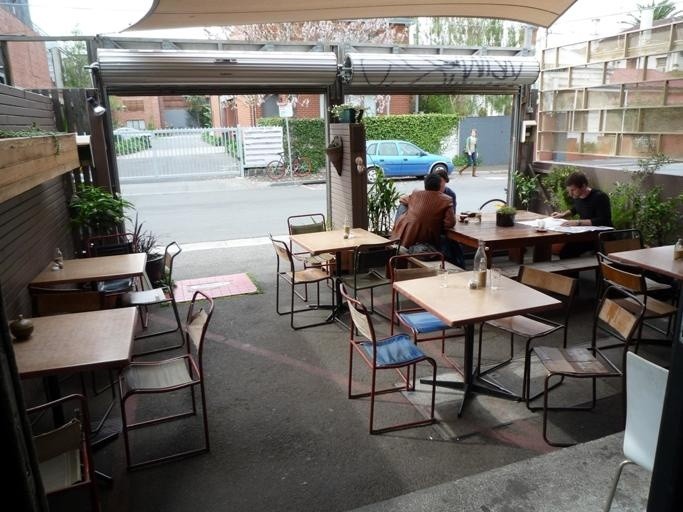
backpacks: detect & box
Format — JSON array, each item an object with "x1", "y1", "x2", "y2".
[{"x1": 409, "y1": 242, "x2": 441, "y2": 262}]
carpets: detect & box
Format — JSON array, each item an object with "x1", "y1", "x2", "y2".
[{"x1": 159, "y1": 270, "x2": 265, "y2": 308}]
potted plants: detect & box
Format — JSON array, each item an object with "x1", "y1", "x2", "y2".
[
  {"x1": 0, "y1": 118, "x2": 82, "y2": 207},
  {"x1": 365, "y1": 153, "x2": 406, "y2": 250},
  {"x1": 326, "y1": 99, "x2": 371, "y2": 125}
]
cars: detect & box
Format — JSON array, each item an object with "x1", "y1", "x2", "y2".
[{"x1": 367, "y1": 140, "x2": 455, "y2": 182}]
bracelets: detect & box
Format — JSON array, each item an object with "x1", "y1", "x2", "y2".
[{"x1": 577, "y1": 220, "x2": 581, "y2": 226}]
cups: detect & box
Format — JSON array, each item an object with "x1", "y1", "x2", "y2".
[
  {"x1": 489, "y1": 267, "x2": 501, "y2": 290},
  {"x1": 474, "y1": 211, "x2": 481, "y2": 225},
  {"x1": 436, "y1": 269, "x2": 448, "y2": 288},
  {"x1": 535, "y1": 219, "x2": 548, "y2": 232}
]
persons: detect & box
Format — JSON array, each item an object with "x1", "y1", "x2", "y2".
[
  {"x1": 424, "y1": 170, "x2": 465, "y2": 270},
  {"x1": 550, "y1": 172, "x2": 615, "y2": 260},
  {"x1": 390, "y1": 175, "x2": 458, "y2": 249},
  {"x1": 458, "y1": 127, "x2": 479, "y2": 179}
]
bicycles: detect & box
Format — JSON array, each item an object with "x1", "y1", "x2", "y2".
[{"x1": 266, "y1": 150, "x2": 313, "y2": 178}]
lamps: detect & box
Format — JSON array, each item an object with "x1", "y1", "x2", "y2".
[{"x1": 85, "y1": 93, "x2": 107, "y2": 117}]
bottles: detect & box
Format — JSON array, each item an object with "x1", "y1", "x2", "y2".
[
  {"x1": 478, "y1": 257, "x2": 487, "y2": 288},
  {"x1": 473, "y1": 238, "x2": 487, "y2": 289},
  {"x1": 344, "y1": 216, "x2": 349, "y2": 235},
  {"x1": 673, "y1": 238, "x2": 682, "y2": 261},
  {"x1": 54, "y1": 247, "x2": 64, "y2": 269}
]
